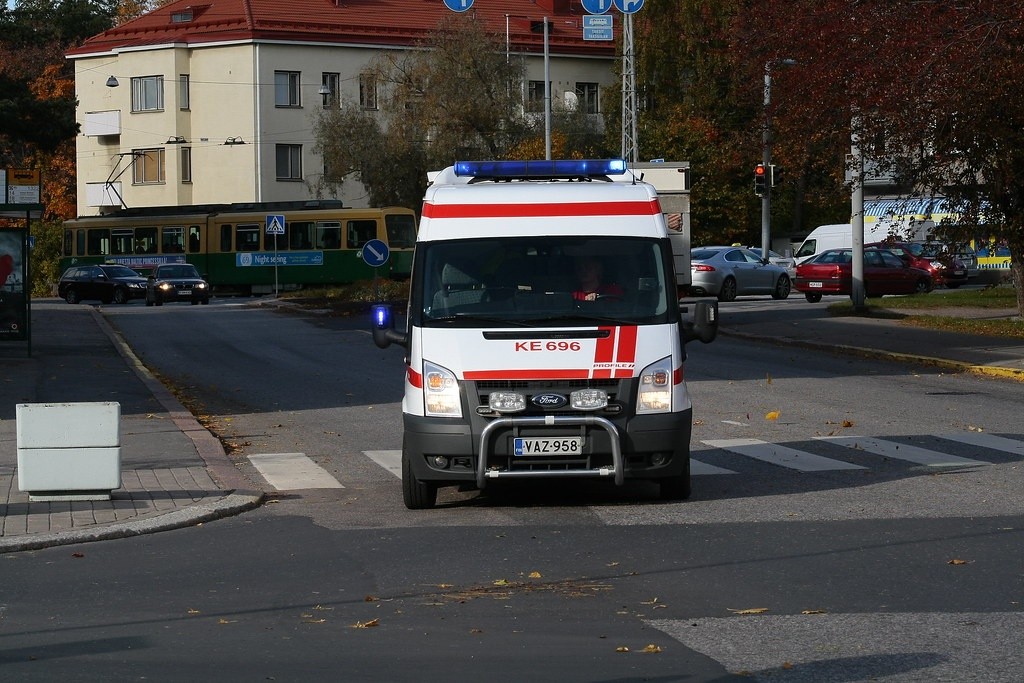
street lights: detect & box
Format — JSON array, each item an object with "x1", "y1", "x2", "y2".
[
  {"x1": 543, "y1": 14, "x2": 574, "y2": 160},
  {"x1": 759, "y1": 56, "x2": 797, "y2": 257},
  {"x1": 505, "y1": 13, "x2": 529, "y2": 119}
]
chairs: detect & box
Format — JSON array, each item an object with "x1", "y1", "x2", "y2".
[{"x1": 432, "y1": 261, "x2": 494, "y2": 314}]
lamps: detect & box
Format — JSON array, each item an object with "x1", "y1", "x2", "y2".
[
  {"x1": 318, "y1": 84, "x2": 331, "y2": 95},
  {"x1": 106, "y1": 76, "x2": 119, "y2": 87}
]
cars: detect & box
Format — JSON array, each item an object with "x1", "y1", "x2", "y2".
[
  {"x1": 145, "y1": 263, "x2": 211, "y2": 307},
  {"x1": 742, "y1": 247, "x2": 793, "y2": 280},
  {"x1": 793, "y1": 247, "x2": 935, "y2": 304},
  {"x1": 690, "y1": 245, "x2": 792, "y2": 303},
  {"x1": 860, "y1": 241, "x2": 979, "y2": 289}
]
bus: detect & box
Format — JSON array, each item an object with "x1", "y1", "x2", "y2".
[{"x1": 55, "y1": 201, "x2": 417, "y2": 298}]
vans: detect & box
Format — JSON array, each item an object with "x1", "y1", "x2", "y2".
[
  {"x1": 785, "y1": 219, "x2": 936, "y2": 269},
  {"x1": 369, "y1": 159, "x2": 718, "y2": 509}
]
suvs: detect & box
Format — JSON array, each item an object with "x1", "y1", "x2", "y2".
[{"x1": 58, "y1": 263, "x2": 149, "y2": 305}]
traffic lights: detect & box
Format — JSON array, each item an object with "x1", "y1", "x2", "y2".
[{"x1": 754, "y1": 165, "x2": 767, "y2": 197}]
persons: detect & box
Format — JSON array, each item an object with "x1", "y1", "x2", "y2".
[
  {"x1": 572, "y1": 253, "x2": 623, "y2": 301},
  {"x1": 112, "y1": 233, "x2": 200, "y2": 255},
  {"x1": 163, "y1": 269, "x2": 194, "y2": 277},
  {"x1": 236, "y1": 226, "x2": 339, "y2": 251}
]
